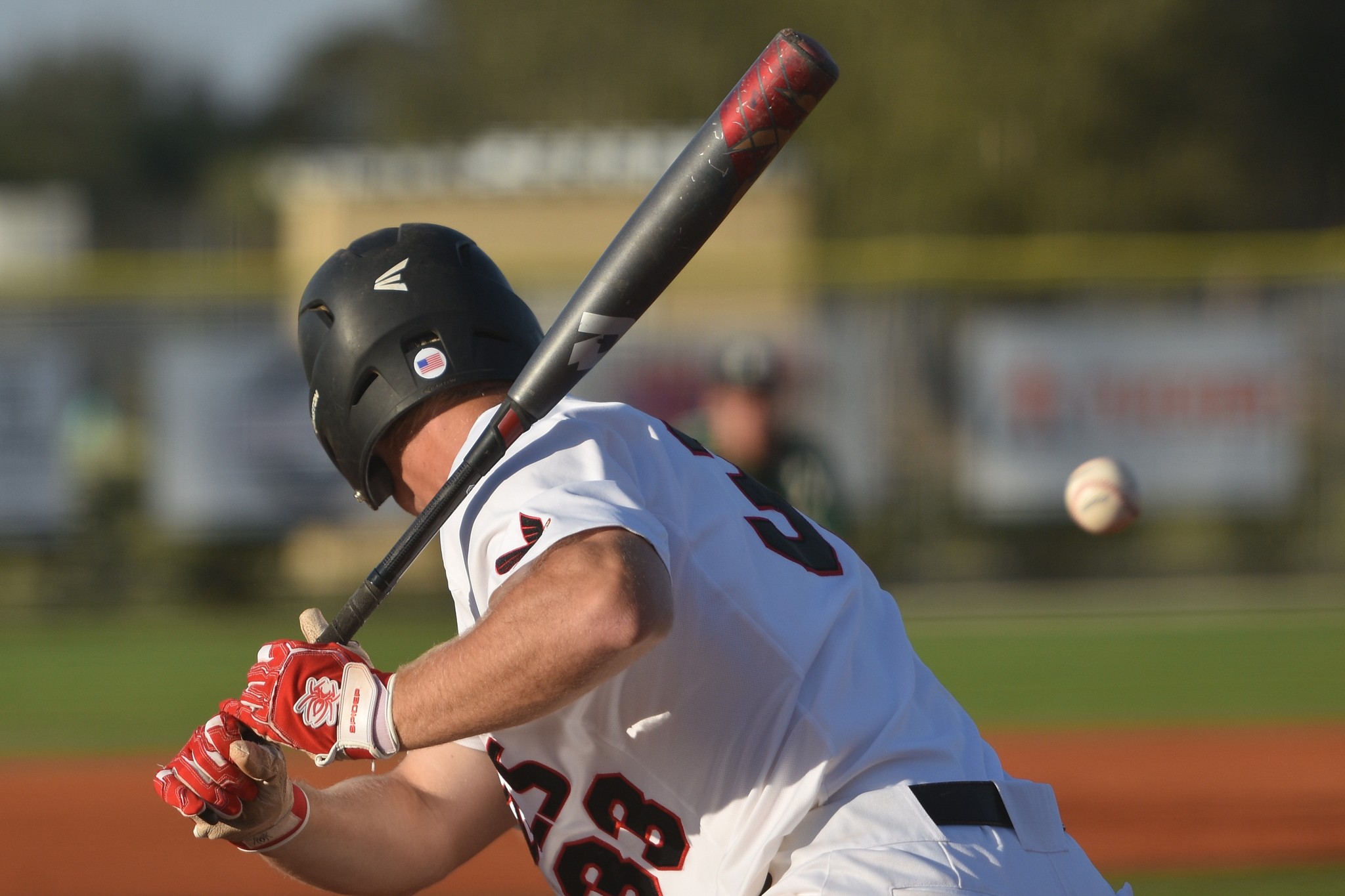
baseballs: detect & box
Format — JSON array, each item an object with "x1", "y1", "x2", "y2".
[{"x1": 1065, "y1": 458, "x2": 1137, "y2": 533}]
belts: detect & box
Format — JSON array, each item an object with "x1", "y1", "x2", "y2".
[{"x1": 909, "y1": 781, "x2": 1010, "y2": 827}]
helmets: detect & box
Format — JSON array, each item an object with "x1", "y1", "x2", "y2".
[{"x1": 298, "y1": 223, "x2": 543, "y2": 512}]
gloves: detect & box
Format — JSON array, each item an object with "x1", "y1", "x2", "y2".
[
  {"x1": 220, "y1": 608, "x2": 400, "y2": 768},
  {"x1": 151, "y1": 711, "x2": 310, "y2": 854}
]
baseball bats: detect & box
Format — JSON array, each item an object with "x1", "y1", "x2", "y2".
[{"x1": 195, "y1": 28, "x2": 840, "y2": 825}]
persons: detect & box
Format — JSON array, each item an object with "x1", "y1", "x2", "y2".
[
  {"x1": 151, "y1": 222, "x2": 1118, "y2": 896},
  {"x1": 51, "y1": 347, "x2": 154, "y2": 620},
  {"x1": 666, "y1": 335, "x2": 851, "y2": 546}
]
